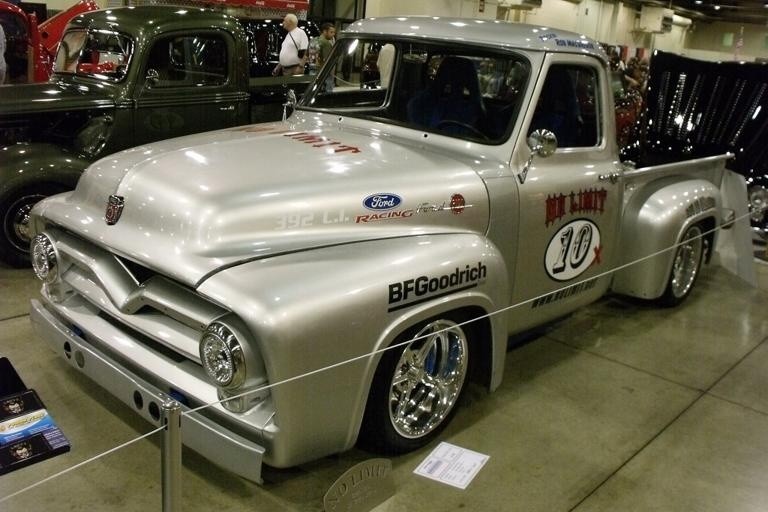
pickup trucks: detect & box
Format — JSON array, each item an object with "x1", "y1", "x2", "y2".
[{"x1": 32, "y1": 17, "x2": 733, "y2": 487}]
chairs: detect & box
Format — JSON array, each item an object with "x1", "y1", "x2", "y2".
[{"x1": 405, "y1": 57, "x2": 580, "y2": 145}]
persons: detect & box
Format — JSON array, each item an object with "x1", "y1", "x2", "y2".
[
  {"x1": 305, "y1": 22, "x2": 337, "y2": 93},
  {"x1": 600, "y1": 43, "x2": 651, "y2": 99},
  {"x1": 270, "y1": 13, "x2": 310, "y2": 77}
]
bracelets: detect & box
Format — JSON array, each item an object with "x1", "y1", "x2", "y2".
[{"x1": 298, "y1": 63, "x2": 304, "y2": 68}]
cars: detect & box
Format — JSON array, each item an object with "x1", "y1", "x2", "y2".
[
  {"x1": 358, "y1": 39, "x2": 767, "y2": 228},
  {"x1": 0, "y1": 1, "x2": 387, "y2": 268}
]
